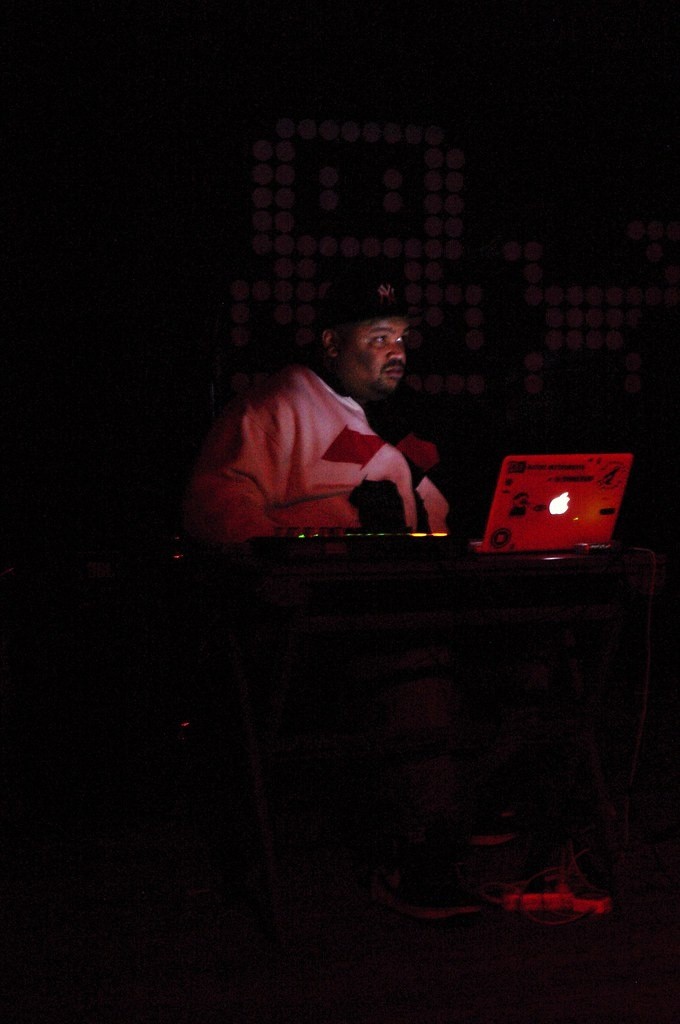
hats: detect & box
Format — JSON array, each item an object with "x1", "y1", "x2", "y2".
[{"x1": 301, "y1": 272, "x2": 418, "y2": 330}]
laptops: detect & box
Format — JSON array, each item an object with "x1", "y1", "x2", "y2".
[{"x1": 469, "y1": 451, "x2": 633, "y2": 553}]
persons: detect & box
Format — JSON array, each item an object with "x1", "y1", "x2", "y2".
[{"x1": 183, "y1": 269, "x2": 607, "y2": 919}]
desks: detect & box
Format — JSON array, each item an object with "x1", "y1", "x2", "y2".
[{"x1": 188, "y1": 533, "x2": 666, "y2": 946}]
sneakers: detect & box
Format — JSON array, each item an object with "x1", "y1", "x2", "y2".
[{"x1": 369, "y1": 845, "x2": 479, "y2": 921}]
